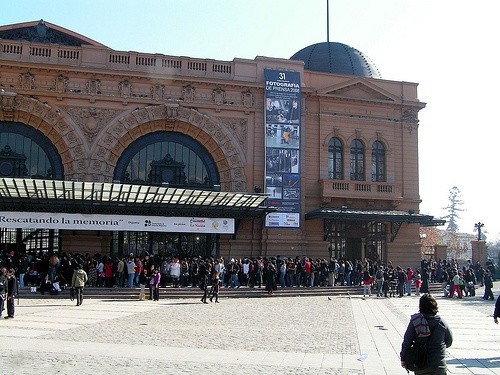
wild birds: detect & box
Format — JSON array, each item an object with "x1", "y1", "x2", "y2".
[{"x1": 37, "y1": 19, "x2": 49, "y2": 41}]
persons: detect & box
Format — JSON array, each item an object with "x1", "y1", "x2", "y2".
[
  {"x1": 0, "y1": 248, "x2": 123, "y2": 295},
  {"x1": 493, "y1": 294, "x2": 500, "y2": 324},
  {"x1": 200, "y1": 266, "x2": 212, "y2": 304},
  {"x1": 209, "y1": 269, "x2": 220, "y2": 303},
  {"x1": 4, "y1": 267, "x2": 19, "y2": 319},
  {"x1": 138, "y1": 269, "x2": 150, "y2": 301},
  {"x1": 441, "y1": 266, "x2": 476, "y2": 298},
  {"x1": 0, "y1": 267, "x2": 8, "y2": 318},
  {"x1": 419, "y1": 258, "x2": 496, "y2": 286},
  {"x1": 148, "y1": 263, "x2": 156, "y2": 300},
  {"x1": 122, "y1": 253, "x2": 351, "y2": 295},
  {"x1": 71, "y1": 264, "x2": 88, "y2": 305},
  {"x1": 153, "y1": 269, "x2": 161, "y2": 301},
  {"x1": 399, "y1": 292, "x2": 453, "y2": 375},
  {"x1": 347, "y1": 257, "x2": 422, "y2": 300},
  {"x1": 482, "y1": 267, "x2": 494, "y2": 299}
]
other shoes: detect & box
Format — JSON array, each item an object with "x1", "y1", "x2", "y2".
[
  {"x1": 4, "y1": 316, "x2": 14, "y2": 319},
  {"x1": 200, "y1": 299, "x2": 208, "y2": 304}
]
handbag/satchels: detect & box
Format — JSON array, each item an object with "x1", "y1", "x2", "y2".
[
  {"x1": 489, "y1": 283, "x2": 493, "y2": 288},
  {"x1": 70, "y1": 288, "x2": 74, "y2": 301},
  {"x1": 404, "y1": 347, "x2": 415, "y2": 371}
]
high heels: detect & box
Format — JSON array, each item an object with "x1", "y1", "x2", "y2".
[
  {"x1": 215, "y1": 301, "x2": 219, "y2": 303},
  {"x1": 209, "y1": 298, "x2": 212, "y2": 302}
]
